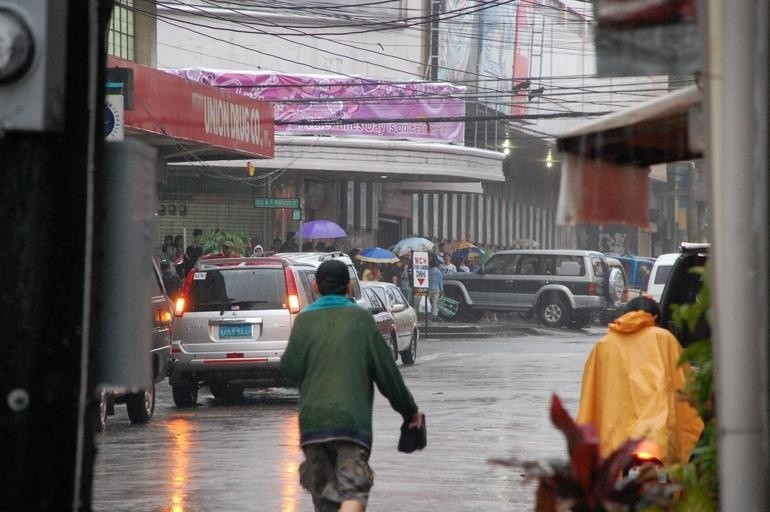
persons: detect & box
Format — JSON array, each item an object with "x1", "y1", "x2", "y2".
[
  {"x1": 250, "y1": 244, "x2": 263, "y2": 261},
  {"x1": 159, "y1": 259, "x2": 182, "y2": 295},
  {"x1": 303, "y1": 242, "x2": 412, "y2": 282},
  {"x1": 172, "y1": 236, "x2": 184, "y2": 264},
  {"x1": 184, "y1": 229, "x2": 203, "y2": 272},
  {"x1": 283, "y1": 232, "x2": 297, "y2": 251},
  {"x1": 273, "y1": 239, "x2": 280, "y2": 254},
  {"x1": 161, "y1": 235, "x2": 177, "y2": 259},
  {"x1": 217, "y1": 242, "x2": 238, "y2": 266},
  {"x1": 580, "y1": 297, "x2": 693, "y2": 463},
  {"x1": 427, "y1": 261, "x2": 444, "y2": 321},
  {"x1": 426, "y1": 242, "x2": 485, "y2": 274},
  {"x1": 279, "y1": 260, "x2": 421, "y2": 512}
]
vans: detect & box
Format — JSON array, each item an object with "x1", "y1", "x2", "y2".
[
  {"x1": 273, "y1": 251, "x2": 366, "y2": 310},
  {"x1": 648, "y1": 252, "x2": 705, "y2": 308},
  {"x1": 594, "y1": 258, "x2": 629, "y2": 324},
  {"x1": 621, "y1": 256, "x2": 655, "y2": 304}
]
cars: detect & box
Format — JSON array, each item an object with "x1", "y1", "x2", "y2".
[
  {"x1": 366, "y1": 281, "x2": 419, "y2": 365},
  {"x1": 359, "y1": 285, "x2": 396, "y2": 370}
]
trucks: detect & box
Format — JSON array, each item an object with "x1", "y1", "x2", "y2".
[{"x1": 96, "y1": 140, "x2": 172, "y2": 422}]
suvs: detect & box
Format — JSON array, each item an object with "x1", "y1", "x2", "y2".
[
  {"x1": 167, "y1": 256, "x2": 328, "y2": 409},
  {"x1": 442, "y1": 248, "x2": 623, "y2": 329}
]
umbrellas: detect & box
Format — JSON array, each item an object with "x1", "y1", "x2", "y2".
[
  {"x1": 392, "y1": 237, "x2": 434, "y2": 255},
  {"x1": 354, "y1": 247, "x2": 399, "y2": 263},
  {"x1": 293, "y1": 220, "x2": 347, "y2": 239},
  {"x1": 448, "y1": 240, "x2": 474, "y2": 250}
]
